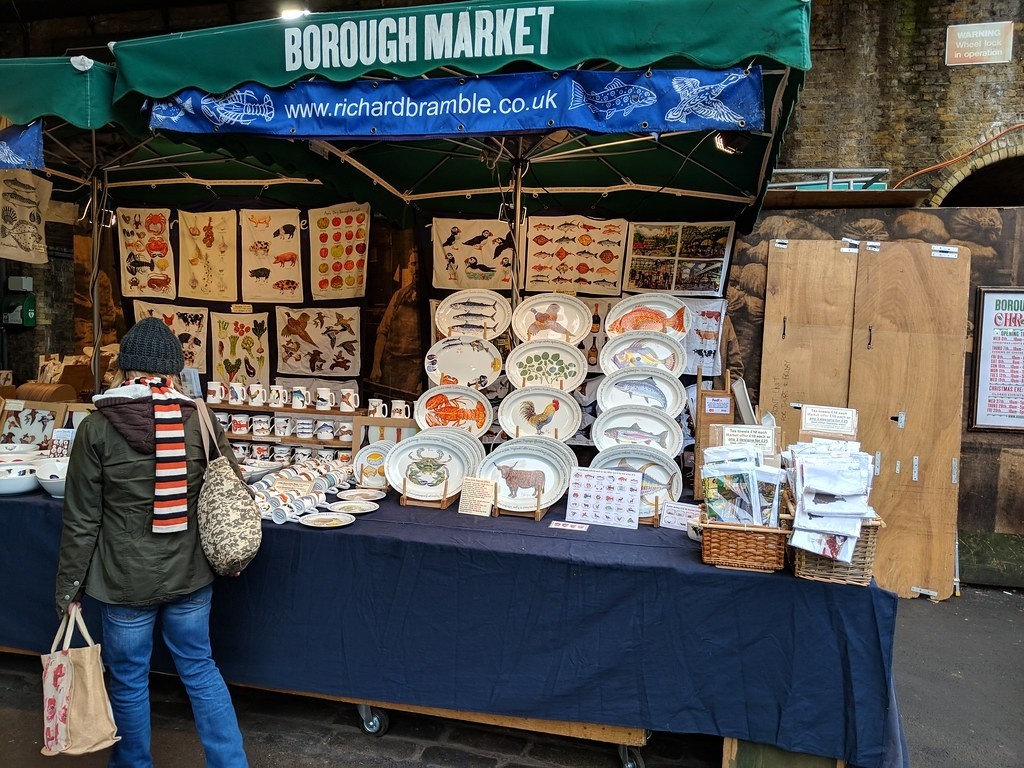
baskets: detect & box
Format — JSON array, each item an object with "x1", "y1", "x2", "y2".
[
  {"x1": 783, "y1": 487, "x2": 887, "y2": 587},
  {"x1": 699, "y1": 502, "x2": 793, "y2": 573}
]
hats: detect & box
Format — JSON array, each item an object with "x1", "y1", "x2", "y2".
[{"x1": 118, "y1": 317, "x2": 184, "y2": 375}]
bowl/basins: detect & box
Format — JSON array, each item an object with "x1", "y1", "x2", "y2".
[{"x1": 1, "y1": 442, "x2": 70, "y2": 499}]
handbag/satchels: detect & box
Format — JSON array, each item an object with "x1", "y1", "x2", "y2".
[
  {"x1": 193, "y1": 397, "x2": 262, "y2": 573},
  {"x1": 40, "y1": 604, "x2": 121, "y2": 756}
]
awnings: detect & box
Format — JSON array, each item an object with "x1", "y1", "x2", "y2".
[{"x1": 0, "y1": 0, "x2": 812, "y2": 398}]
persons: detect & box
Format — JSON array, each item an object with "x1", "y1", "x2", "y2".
[
  {"x1": 371, "y1": 249, "x2": 424, "y2": 404},
  {"x1": 53, "y1": 317, "x2": 251, "y2": 768}
]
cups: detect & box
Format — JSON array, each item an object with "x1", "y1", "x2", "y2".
[
  {"x1": 252, "y1": 415, "x2": 275, "y2": 436},
  {"x1": 391, "y1": 400, "x2": 411, "y2": 419},
  {"x1": 291, "y1": 418, "x2": 314, "y2": 438},
  {"x1": 269, "y1": 385, "x2": 288, "y2": 408},
  {"x1": 315, "y1": 387, "x2": 335, "y2": 411},
  {"x1": 274, "y1": 416, "x2": 296, "y2": 437},
  {"x1": 339, "y1": 388, "x2": 359, "y2": 413},
  {"x1": 214, "y1": 412, "x2": 233, "y2": 433},
  {"x1": 228, "y1": 383, "x2": 246, "y2": 405},
  {"x1": 248, "y1": 383, "x2": 267, "y2": 407},
  {"x1": 313, "y1": 419, "x2": 335, "y2": 440},
  {"x1": 230, "y1": 442, "x2": 358, "y2": 524},
  {"x1": 206, "y1": 382, "x2": 225, "y2": 404},
  {"x1": 367, "y1": 398, "x2": 388, "y2": 417},
  {"x1": 334, "y1": 421, "x2": 353, "y2": 442},
  {"x1": 231, "y1": 415, "x2": 253, "y2": 435},
  {"x1": 292, "y1": 386, "x2": 311, "y2": 410}
]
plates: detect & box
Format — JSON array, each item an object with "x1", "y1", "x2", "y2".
[{"x1": 299, "y1": 287, "x2": 692, "y2": 528}]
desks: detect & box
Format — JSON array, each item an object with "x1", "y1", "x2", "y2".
[{"x1": 0, "y1": 490, "x2": 909, "y2": 768}]
